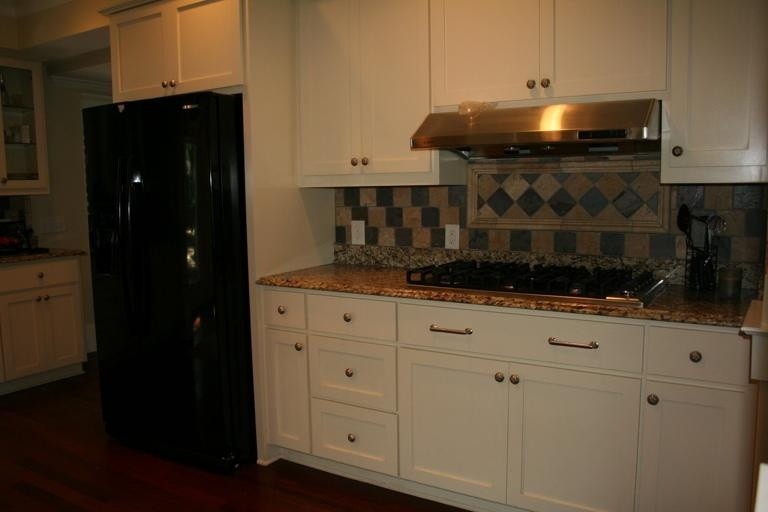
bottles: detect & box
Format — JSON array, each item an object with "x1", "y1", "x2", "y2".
[
  {"x1": 717, "y1": 263, "x2": 743, "y2": 300},
  {"x1": 0, "y1": 71, "x2": 8, "y2": 104}
]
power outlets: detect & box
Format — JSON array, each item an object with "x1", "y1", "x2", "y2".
[
  {"x1": 350, "y1": 220, "x2": 366, "y2": 245},
  {"x1": 443, "y1": 223, "x2": 460, "y2": 250}
]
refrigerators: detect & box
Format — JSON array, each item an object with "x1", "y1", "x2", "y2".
[{"x1": 81, "y1": 91, "x2": 258, "y2": 466}]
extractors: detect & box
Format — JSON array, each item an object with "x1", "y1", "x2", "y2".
[{"x1": 409, "y1": 98, "x2": 661, "y2": 162}]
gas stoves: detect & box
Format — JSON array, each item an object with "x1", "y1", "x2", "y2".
[{"x1": 407, "y1": 259, "x2": 672, "y2": 309}]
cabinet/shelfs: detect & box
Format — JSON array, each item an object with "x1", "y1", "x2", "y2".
[
  {"x1": 253, "y1": 280, "x2": 764, "y2": 512},
  {"x1": 1, "y1": 53, "x2": 87, "y2": 387},
  {"x1": 99, "y1": 0, "x2": 767, "y2": 188}
]
